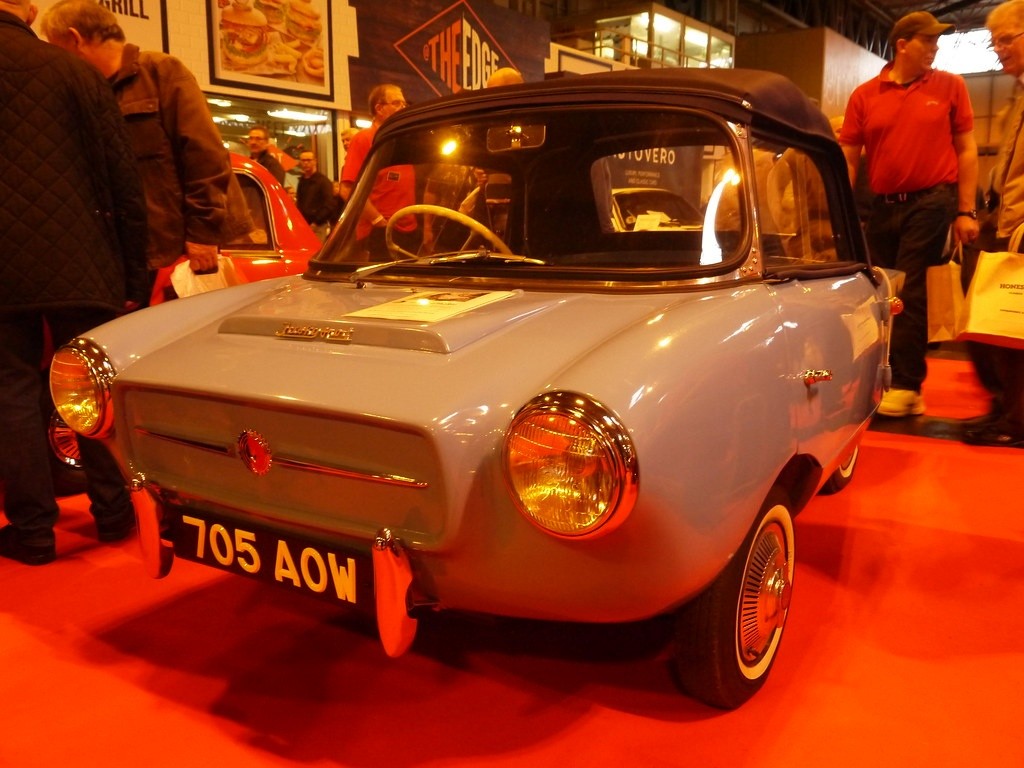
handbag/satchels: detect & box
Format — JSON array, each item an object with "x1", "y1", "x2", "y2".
[
  {"x1": 149, "y1": 253, "x2": 241, "y2": 306},
  {"x1": 218, "y1": 171, "x2": 256, "y2": 243},
  {"x1": 927, "y1": 222, "x2": 966, "y2": 342},
  {"x1": 955, "y1": 222, "x2": 1024, "y2": 350}
]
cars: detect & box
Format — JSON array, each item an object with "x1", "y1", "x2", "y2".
[
  {"x1": 141, "y1": 151, "x2": 326, "y2": 315},
  {"x1": 45, "y1": 61, "x2": 894, "y2": 712},
  {"x1": 607, "y1": 185, "x2": 711, "y2": 232}
]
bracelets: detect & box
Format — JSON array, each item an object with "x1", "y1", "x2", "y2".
[{"x1": 372, "y1": 215, "x2": 383, "y2": 225}]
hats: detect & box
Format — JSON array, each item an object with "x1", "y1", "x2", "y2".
[{"x1": 888, "y1": 11, "x2": 956, "y2": 47}]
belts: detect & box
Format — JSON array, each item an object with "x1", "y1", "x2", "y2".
[{"x1": 877, "y1": 183, "x2": 946, "y2": 204}]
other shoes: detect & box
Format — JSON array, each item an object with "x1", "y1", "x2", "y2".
[
  {"x1": 96, "y1": 502, "x2": 135, "y2": 540},
  {"x1": 0, "y1": 524, "x2": 55, "y2": 564},
  {"x1": 961, "y1": 413, "x2": 1001, "y2": 428},
  {"x1": 964, "y1": 422, "x2": 1024, "y2": 447}
]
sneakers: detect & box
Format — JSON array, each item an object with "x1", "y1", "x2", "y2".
[{"x1": 878, "y1": 390, "x2": 926, "y2": 417}]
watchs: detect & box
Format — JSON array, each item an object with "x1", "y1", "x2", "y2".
[{"x1": 958, "y1": 209, "x2": 976, "y2": 218}]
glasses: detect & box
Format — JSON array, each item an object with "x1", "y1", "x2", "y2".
[
  {"x1": 987, "y1": 31, "x2": 1024, "y2": 51},
  {"x1": 301, "y1": 159, "x2": 315, "y2": 163},
  {"x1": 381, "y1": 101, "x2": 408, "y2": 109}
]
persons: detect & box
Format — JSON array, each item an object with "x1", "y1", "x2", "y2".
[
  {"x1": 297, "y1": 151, "x2": 332, "y2": 244},
  {"x1": 962, "y1": 1, "x2": 1024, "y2": 446},
  {"x1": 839, "y1": 10, "x2": 979, "y2": 414},
  {"x1": 0, "y1": 0, "x2": 149, "y2": 565},
  {"x1": 339, "y1": 83, "x2": 420, "y2": 262},
  {"x1": 248, "y1": 125, "x2": 285, "y2": 188},
  {"x1": 425, "y1": 68, "x2": 615, "y2": 252},
  {"x1": 41, "y1": 0, "x2": 257, "y2": 309},
  {"x1": 713, "y1": 116, "x2": 843, "y2": 237}
]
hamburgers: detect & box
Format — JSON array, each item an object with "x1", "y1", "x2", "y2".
[{"x1": 218, "y1": 0, "x2": 322, "y2": 66}]
